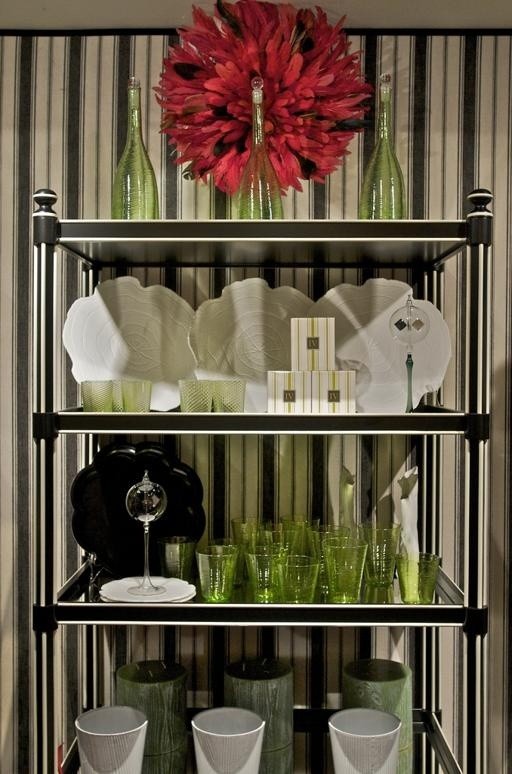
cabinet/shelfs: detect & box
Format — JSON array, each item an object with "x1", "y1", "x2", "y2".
[{"x1": 33, "y1": 188, "x2": 494, "y2": 774}]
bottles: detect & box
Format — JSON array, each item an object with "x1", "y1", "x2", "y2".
[
  {"x1": 358, "y1": 73, "x2": 406, "y2": 218},
  {"x1": 110, "y1": 77, "x2": 160, "y2": 219},
  {"x1": 238, "y1": 76, "x2": 283, "y2": 220}
]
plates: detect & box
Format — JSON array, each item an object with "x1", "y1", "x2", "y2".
[{"x1": 99, "y1": 575, "x2": 197, "y2": 603}]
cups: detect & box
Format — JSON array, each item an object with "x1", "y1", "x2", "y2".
[
  {"x1": 75, "y1": 706, "x2": 148, "y2": 773},
  {"x1": 191, "y1": 707, "x2": 266, "y2": 774},
  {"x1": 195, "y1": 546, "x2": 240, "y2": 602},
  {"x1": 244, "y1": 546, "x2": 289, "y2": 604},
  {"x1": 259, "y1": 524, "x2": 303, "y2": 555},
  {"x1": 307, "y1": 525, "x2": 351, "y2": 593},
  {"x1": 232, "y1": 518, "x2": 272, "y2": 578},
  {"x1": 395, "y1": 553, "x2": 441, "y2": 606},
  {"x1": 81, "y1": 381, "x2": 112, "y2": 412},
  {"x1": 179, "y1": 380, "x2": 213, "y2": 412},
  {"x1": 222, "y1": 660, "x2": 295, "y2": 773},
  {"x1": 321, "y1": 537, "x2": 369, "y2": 603},
  {"x1": 157, "y1": 536, "x2": 194, "y2": 584},
  {"x1": 357, "y1": 523, "x2": 402, "y2": 585},
  {"x1": 206, "y1": 538, "x2": 248, "y2": 588},
  {"x1": 328, "y1": 707, "x2": 401, "y2": 774},
  {"x1": 111, "y1": 379, "x2": 126, "y2": 411},
  {"x1": 344, "y1": 660, "x2": 412, "y2": 774},
  {"x1": 275, "y1": 555, "x2": 320, "y2": 604},
  {"x1": 123, "y1": 380, "x2": 153, "y2": 411},
  {"x1": 281, "y1": 515, "x2": 320, "y2": 563},
  {"x1": 213, "y1": 380, "x2": 247, "y2": 411},
  {"x1": 116, "y1": 661, "x2": 188, "y2": 774}
]
